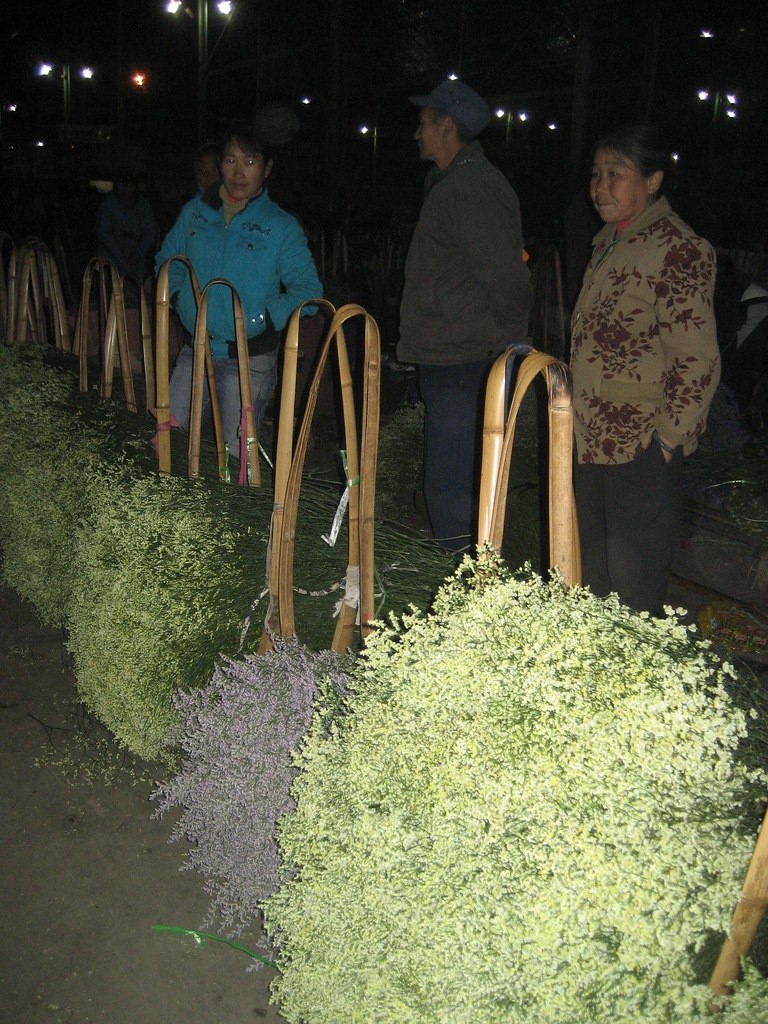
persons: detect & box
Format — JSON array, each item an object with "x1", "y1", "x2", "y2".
[{"x1": 94, "y1": 80, "x2": 722, "y2": 618}]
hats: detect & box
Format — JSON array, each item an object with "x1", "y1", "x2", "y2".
[{"x1": 409, "y1": 79, "x2": 491, "y2": 136}]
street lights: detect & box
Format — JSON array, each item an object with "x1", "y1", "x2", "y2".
[
  {"x1": 34, "y1": 63, "x2": 94, "y2": 128},
  {"x1": 132, "y1": 72, "x2": 153, "y2": 143},
  {"x1": 695, "y1": 88, "x2": 741, "y2": 129},
  {"x1": 161, "y1": 0, "x2": 237, "y2": 159},
  {"x1": 493, "y1": 109, "x2": 530, "y2": 145},
  {"x1": 358, "y1": 124, "x2": 377, "y2": 217}
]
